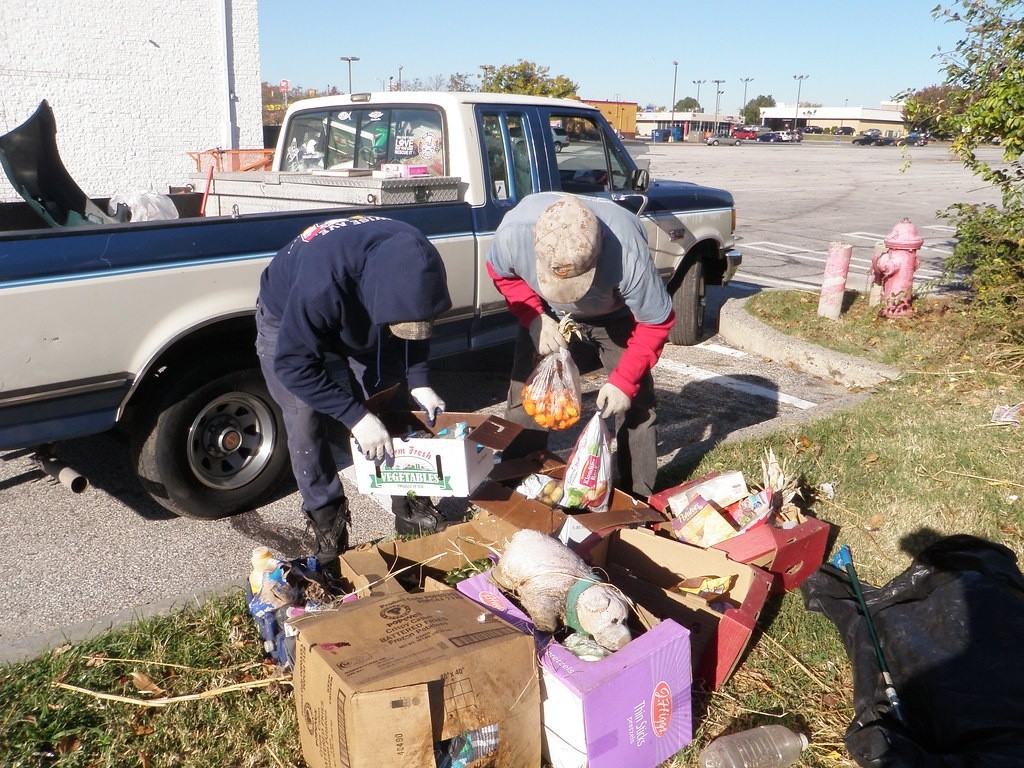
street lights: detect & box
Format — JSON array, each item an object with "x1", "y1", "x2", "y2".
[
  {"x1": 399, "y1": 65, "x2": 404, "y2": 90},
  {"x1": 693, "y1": 80, "x2": 707, "y2": 111},
  {"x1": 793, "y1": 75, "x2": 809, "y2": 129},
  {"x1": 739, "y1": 78, "x2": 754, "y2": 125},
  {"x1": 966, "y1": 26, "x2": 1003, "y2": 111},
  {"x1": 389, "y1": 75, "x2": 394, "y2": 90},
  {"x1": 341, "y1": 56, "x2": 360, "y2": 95},
  {"x1": 668, "y1": 60, "x2": 679, "y2": 143},
  {"x1": 713, "y1": 80, "x2": 726, "y2": 134}
]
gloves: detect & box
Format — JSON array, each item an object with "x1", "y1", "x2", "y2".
[
  {"x1": 410, "y1": 387, "x2": 445, "y2": 427},
  {"x1": 351, "y1": 412, "x2": 395, "y2": 467},
  {"x1": 596, "y1": 383, "x2": 631, "y2": 419},
  {"x1": 528, "y1": 313, "x2": 568, "y2": 355}
]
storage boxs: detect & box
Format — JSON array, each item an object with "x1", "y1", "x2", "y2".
[
  {"x1": 349, "y1": 380, "x2": 525, "y2": 496},
  {"x1": 337, "y1": 509, "x2": 523, "y2": 599},
  {"x1": 245, "y1": 541, "x2": 407, "y2": 663},
  {"x1": 460, "y1": 562, "x2": 692, "y2": 768},
  {"x1": 646, "y1": 470, "x2": 831, "y2": 601},
  {"x1": 278, "y1": 574, "x2": 542, "y2": 767},
  {"x1": 572, "y1": 528, "x2": 777, "y2": 690},
  {"x1": 467, "y1": 448, "x2": 667, "y2": 569}
]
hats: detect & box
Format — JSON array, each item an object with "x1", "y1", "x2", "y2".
[
  {"x1": 389, "y1": 316, "x2": 434, "y2": 340},
  {"x1": 535, "y1": 195, "x2": 600, "y2": 303}
]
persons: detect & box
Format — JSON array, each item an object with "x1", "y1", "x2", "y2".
[
  {"x1": 252, "y1": 216, "x2": 452, "y2": 576},
  {"x1": 484, "y1": 187, "x2": 677, "y2": 503}
]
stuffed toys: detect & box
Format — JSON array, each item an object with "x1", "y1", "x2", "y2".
[{"x1": 493, "y1": 526, "x2": 632, "y2": 650}]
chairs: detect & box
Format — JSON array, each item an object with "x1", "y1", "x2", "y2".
[{"x1": 512, "y1": 141, "x2": 531, "y2": 198}]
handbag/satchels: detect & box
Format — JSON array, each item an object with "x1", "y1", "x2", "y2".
[
  {"x1": 521, "y1": 347, "x2": 581, "y2": 430},
  {"x1": 799, "y1": 535, "x2": 1024, "y2": 768}
]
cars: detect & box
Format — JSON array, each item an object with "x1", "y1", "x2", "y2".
[
  {"x1": 796, "y1": 126, "x2": 823, "y2": 134},
  {"x1": 732, "y1": 129, "x2": 757, "y2": 140},
  {"x1": 756, "y1": 131, "x2": 804, "y2": 143},
  {"x1": 852, "y1": 129, "x2": 928, "y2": 147},
  {"x1": 707, "y1": 134, "x2": 742, "y2": 146},
  {"x1": 743, "y1": 125, "x2": 772, "y2": 132}
]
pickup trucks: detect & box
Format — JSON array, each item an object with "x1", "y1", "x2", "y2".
[{"x1": 0, "y1": 91, "x2": 744, "y2": 517}]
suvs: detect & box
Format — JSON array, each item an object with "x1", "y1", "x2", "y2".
[
  {"x1": 510, "y1": 126, "x2": 570, "y2": 153},
  {"x1": 833, "y1": 126, "x2": 854, "y2": 135}
]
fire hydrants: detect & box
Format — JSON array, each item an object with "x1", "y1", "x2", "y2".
[{"x1": 874, "y1": 216, "x2": 924, "y2": 320}]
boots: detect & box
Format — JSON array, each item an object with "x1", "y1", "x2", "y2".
[
  {"x1": 391, "y1": 495, "x2": 463, "y2": 536},
  {"x1": 301, "y1": 498, "x2": 351, "y2": 555}
]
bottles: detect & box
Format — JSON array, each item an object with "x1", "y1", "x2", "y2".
[
  {"x1": 249, "y1": 547, "x2": 278, "y2": 596},
  {"x1": 697, "y1": 725, "x2": 808, "y2": 768}
]
